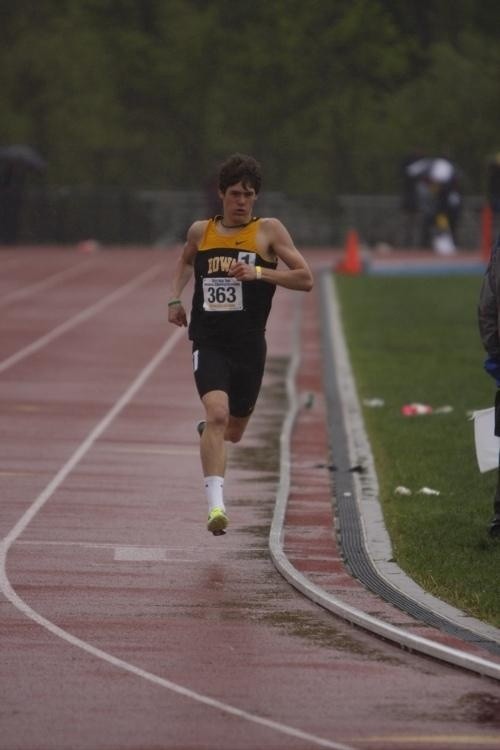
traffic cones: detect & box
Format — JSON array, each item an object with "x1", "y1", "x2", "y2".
[{"x1": 334, "y1": 229, "x2": 362, "y2": 273}]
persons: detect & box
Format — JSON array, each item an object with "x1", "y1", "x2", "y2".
[
  {"x1": 166, "y1": 152, "x2": 315, "y2": 536},
  {"x1": 477, "y1": 214, "x2": 500, "y2": 536}
]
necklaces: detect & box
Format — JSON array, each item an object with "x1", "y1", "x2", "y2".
[{"x1": 220, "y1": 219, "x2": 249, "y2": 228}]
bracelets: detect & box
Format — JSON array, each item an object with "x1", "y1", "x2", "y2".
[
  {"x1": 255, "y1": 266, "x2": 261, "y2": 281},
  {"x1": 168, "y1": 300, "x2": 181, "y2": 306}
]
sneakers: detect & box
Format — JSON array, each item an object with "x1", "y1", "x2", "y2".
[
  {"x1": 207, "y1": 508, "x2": 228, "y2": 536},
  {"x1": 198, "y1": 422, "x2": 206, "y2": 435},
  {"x1": 481, "y1": 523, "x2": 500, "y2": 551}
]
